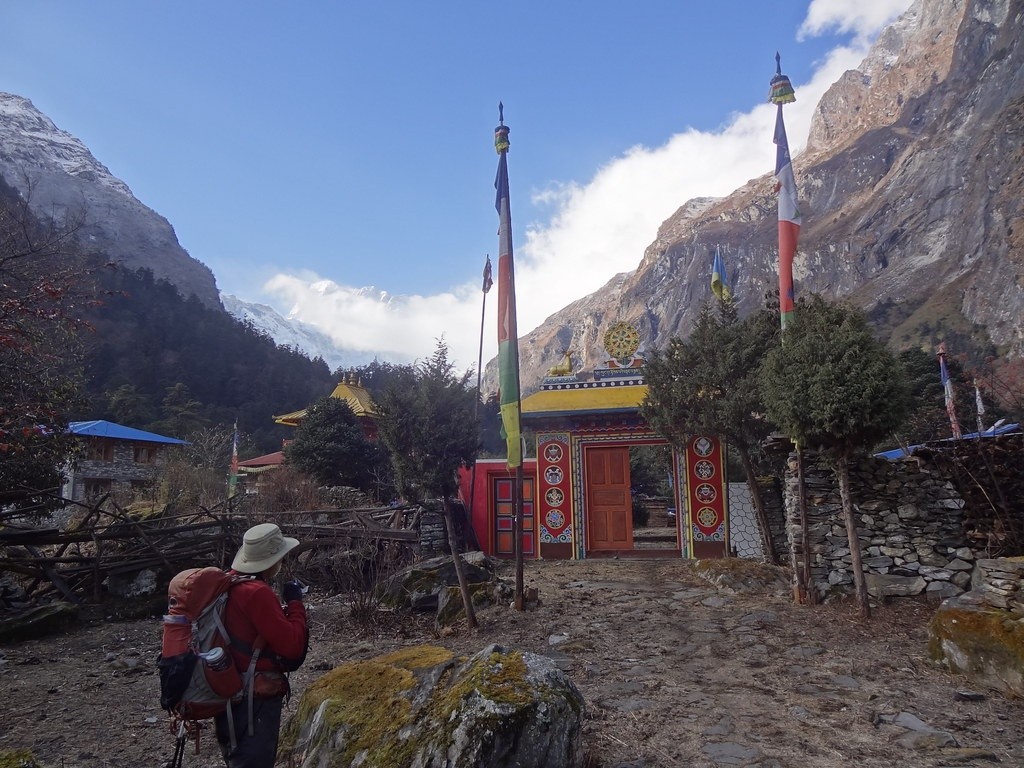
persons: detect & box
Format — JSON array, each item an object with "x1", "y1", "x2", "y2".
[{"x1": 210, "y1": 523, "x2": 310, "y2": 768}]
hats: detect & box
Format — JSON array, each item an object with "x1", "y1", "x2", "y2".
[{"x1": 229, "y1": 521, "x2": 301, "y2": 574}]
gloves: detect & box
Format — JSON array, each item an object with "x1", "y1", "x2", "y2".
[{"x1": 282, "y1": 579, "x2": 301, "y2": 603}]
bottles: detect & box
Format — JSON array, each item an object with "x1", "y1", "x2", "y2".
[{"x1": 198, "y1": 646, "x2": 231, "y2": 672}]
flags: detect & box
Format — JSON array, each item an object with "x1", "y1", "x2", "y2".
[{"x1": 482, "y1": 257, "x2": 493, "y2": 294}]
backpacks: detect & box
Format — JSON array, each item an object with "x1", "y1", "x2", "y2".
[{"x1": 155, "y1": 565, "x2": 270, "y2": 755}]
